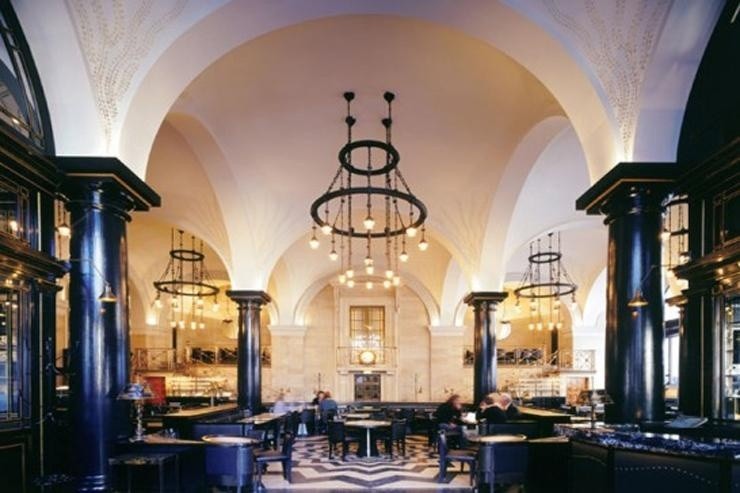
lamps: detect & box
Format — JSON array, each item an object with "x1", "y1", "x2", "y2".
[
  {"x1": 153, "y1": 229, "x2": 220, "y2": 330},
  {"x1": 513, "y1": 231, "x2": 578, "y2": 332},
  {"x1": 309, "y1": 90, "x2": 430, "y2": 289},
  {"x1": 625, "y1": 261, "x2": 659, "y2": 309},
  {"x1": 67, "y1": 256, "x2": 119, "y2": 303}
]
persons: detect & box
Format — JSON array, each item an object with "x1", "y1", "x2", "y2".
[
  {"x1": 432, "y1": 391, "x2": 520, "y2": 456},
  {"x1": 319, "y1": 392, "x2": 338, "y2": 434},
  {"x1": 313, "y1": 391, "x2": 326, "y2": 402}
]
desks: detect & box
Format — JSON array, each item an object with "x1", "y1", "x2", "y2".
[{"x1": 517, "y1": 406, "x2": 575, "y2": 434}]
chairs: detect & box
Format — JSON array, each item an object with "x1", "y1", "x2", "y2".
[
  {"x1": 295, "y1": 404, "x2": 425, "y2": 460},
  {"x1": 426, "y1": 411, "x2": 529, "y2": 491},
  {"x1": 464, "y1": 347, "x2": 543, "y2": 366},
  {"x1": 193, "y1": 346, "x2": 271, "y2": 368},
  {"x1": 106, "y1": 388, "x2": 295, "y2": 492}
]
